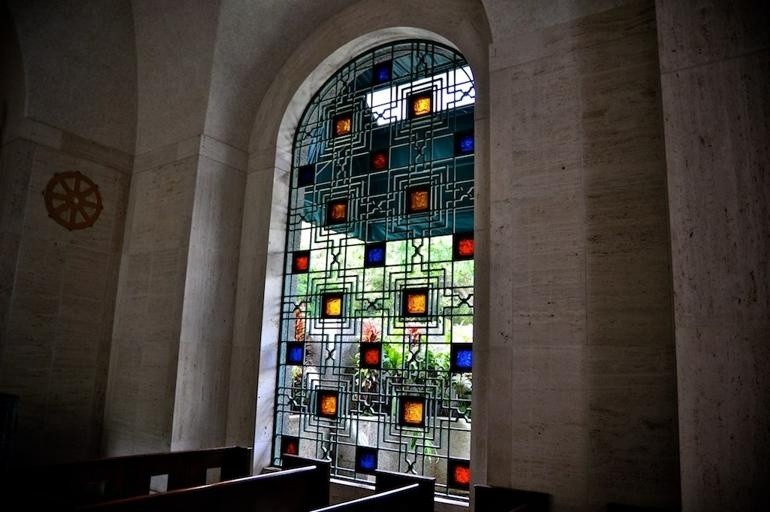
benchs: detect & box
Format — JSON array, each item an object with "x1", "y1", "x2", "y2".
[{"x1": 1, "y1": 446, "x2": 552, "y2": 512}]
